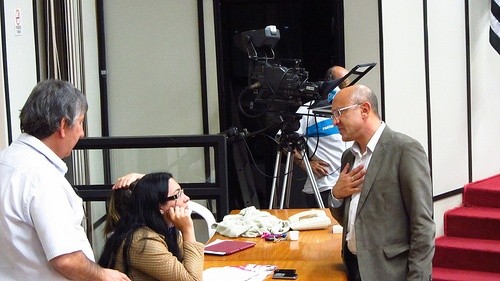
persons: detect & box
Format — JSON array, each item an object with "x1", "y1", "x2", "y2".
[
  {"x1": 0, "y1": 80, "x2": 132, "y2": 281},
  {"x1": 98, "y1": 172, "x2": 205, "y2": 281},
  {"x1": 276, "y1": 66, "x2": 351, "y2": 208},
  {"x1": 328, "y1": 85, "x2": 436, "y2": 281},
  {"x1": 102, "y1": 173, "x2": 146, "y2": 239}
]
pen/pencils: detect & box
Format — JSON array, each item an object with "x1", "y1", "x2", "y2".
[{"x1": 172, "y1": 199, "x2": 177, "y2": 224}]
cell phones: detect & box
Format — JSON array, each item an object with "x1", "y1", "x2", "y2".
[
  {"x1": 272, "y1": 273, "x2": 298, "y2": 279},
  {"x1": 274, "y1": 269, "x2": 296, "y2": 274}
]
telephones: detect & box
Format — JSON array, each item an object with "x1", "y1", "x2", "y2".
[{"x1": 288, "y1": 208, "x2": 333, "y2": 232}]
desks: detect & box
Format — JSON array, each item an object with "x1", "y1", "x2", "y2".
[{"x1": 204, "y1": 208, "x2": 350, "y2": 281}]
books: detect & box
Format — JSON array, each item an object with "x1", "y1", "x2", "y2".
[{"x1": 204, "y1": 239, "x2": 256, "y2": 256}]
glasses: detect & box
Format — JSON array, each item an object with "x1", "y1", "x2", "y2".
[
  {"x1": 162, "y1": 188, "x2": 184, "y2": 202},
  {"x1": 331, "y1": 104, "x2": 362, "y2": 120}
]
